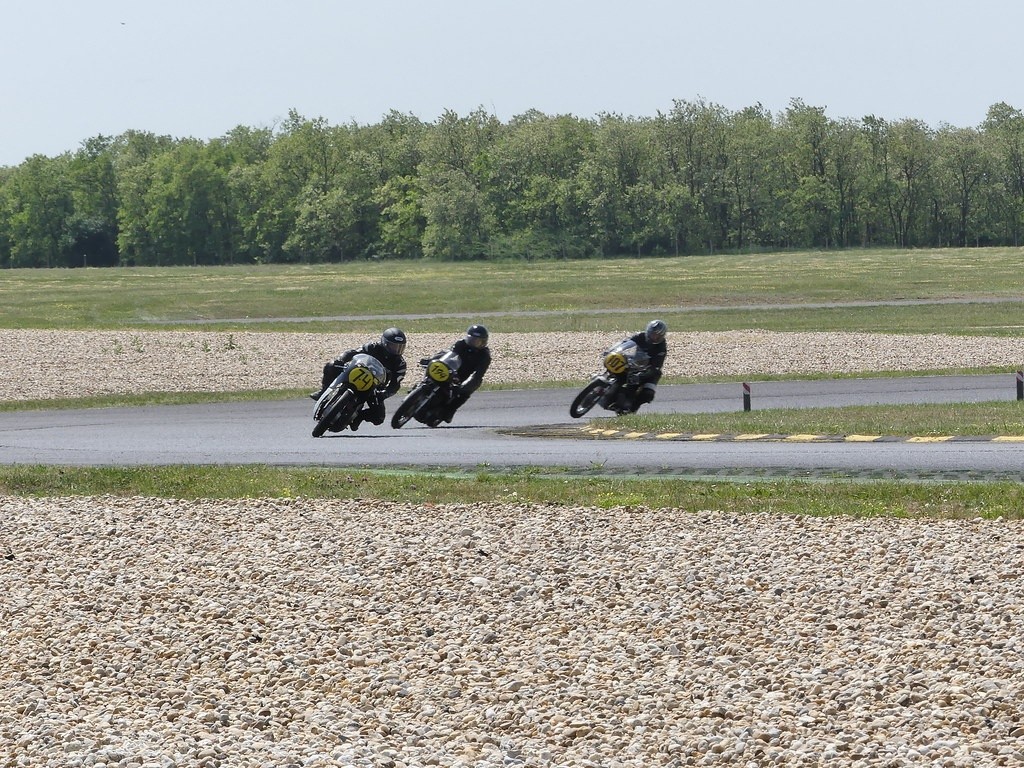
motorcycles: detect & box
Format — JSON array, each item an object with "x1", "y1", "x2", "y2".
[
  {"x1": 312, "y1": 349, "x2": 386, "y2": 438},
  {"x1": 391, "y1": 349, "x2": 484, "y2": 429},
  {"x1": 569, "y1": 338, "x2": 657, "y2": 419}
]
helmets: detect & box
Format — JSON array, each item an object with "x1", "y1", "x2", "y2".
[
  {"x1": 381, "y1": 328, "x2": 407, "y2": 356},
  {"x1": 645, "y1": 320, "x2": 667, "y2": 344},
  {"x1": 465, "y1": 324, "x2": 489, "y2": 348}
]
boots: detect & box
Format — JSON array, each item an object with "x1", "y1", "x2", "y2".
[
  {"x1": 350, "y1": 415, "x2": 364, "y2": 431},
  {"x1": 310, "y1": 374, "x2": 334, "y2": 401}
]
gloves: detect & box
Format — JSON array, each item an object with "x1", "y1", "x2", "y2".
[
  {"x1": 333, "y1": 360, "x2": 344, "y2": 367},
  {"x1": 377, "y1": 391, "x2": 387, "y2": 401},
  {"x1": 420, "y1": 357, "x2": 431, "y2": 365},
  {"x1": 450, "y1": 383, "x2": 460, "y2": 391}
]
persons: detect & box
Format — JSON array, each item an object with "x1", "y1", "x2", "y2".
[
  {"x1": 600, "y1": 319, "x2": 667, "y2": 411},
  {"x1": 420, "y1": 324, "x2": 491, "y2": 423},
  {"x1": 309, "y1": 328, "x2": 407, "y2": 432}
]
